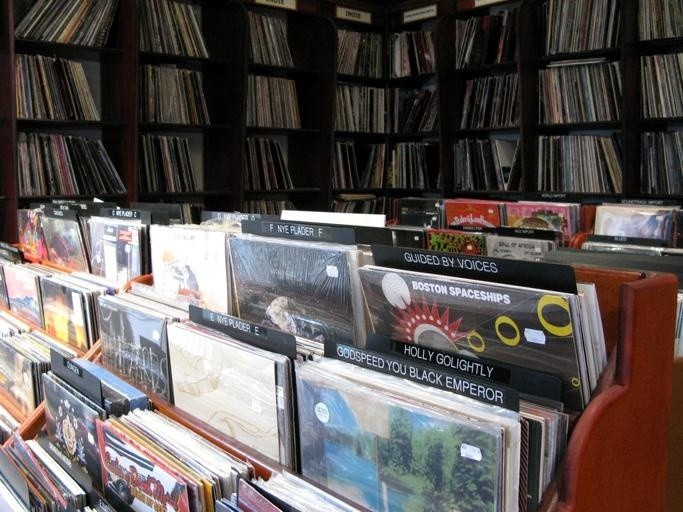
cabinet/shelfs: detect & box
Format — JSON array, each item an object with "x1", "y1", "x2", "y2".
[{"x1": 1, "y1": 3, "x2": 680, "y2": 511}]
